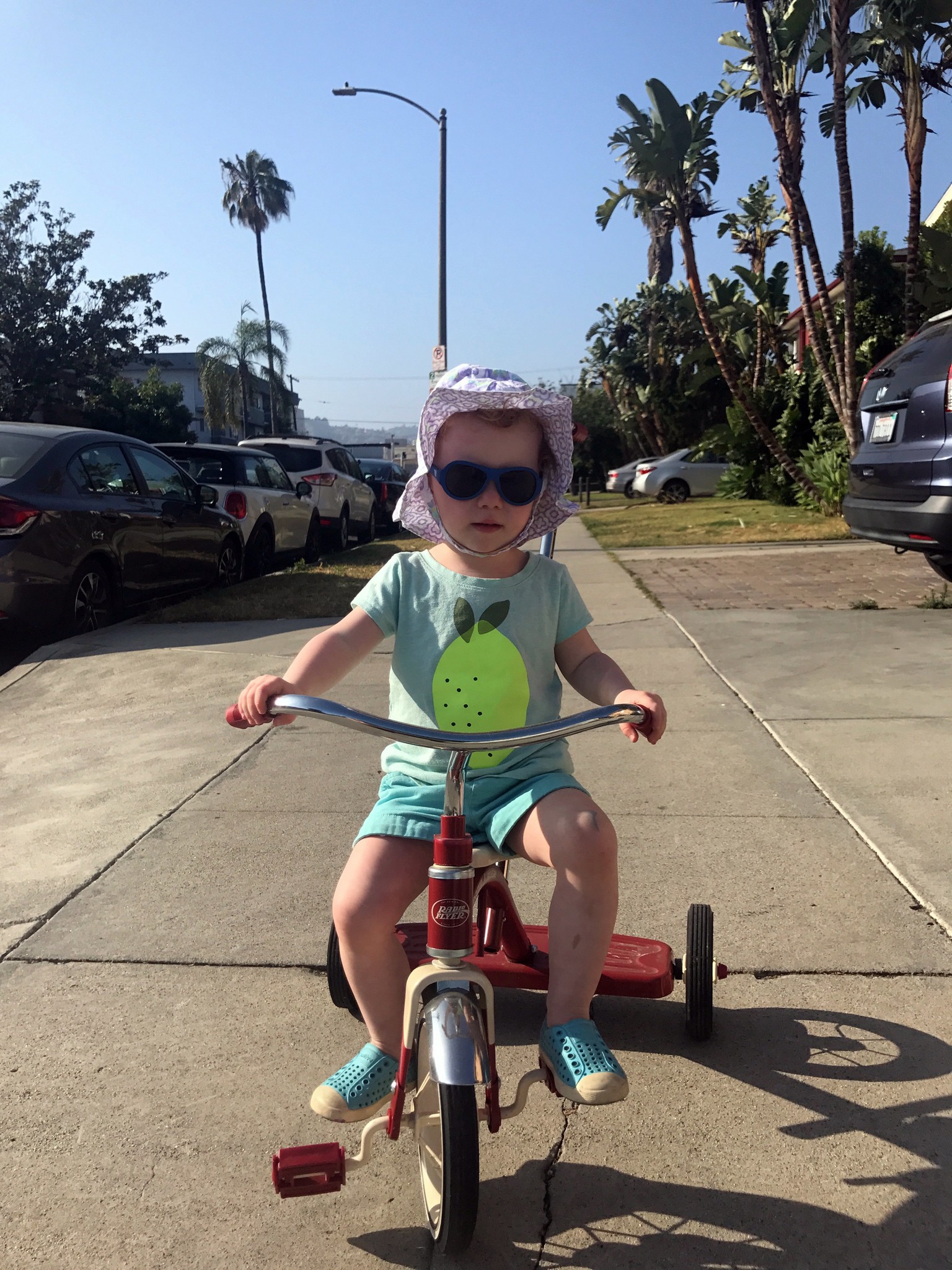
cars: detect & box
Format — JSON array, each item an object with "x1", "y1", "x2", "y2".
[
  {"x1": 355, "y1": 457, "x2": 410, "y2": 534},
  {"x1": 107, "y1": 441, "x2": 321, "y2": 578},
  {"x1": 0, "y1": 421, "x2": 247, "y2": 637},
  {"x1": 605, "y1": 456, "x2": 665, "y2": 500},
  {"x1": 632, "y1": 447, "x2": 766, "y2": 504}
]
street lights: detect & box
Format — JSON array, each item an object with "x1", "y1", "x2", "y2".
[{"x1": 331, "y1": 82, "x2": 449, "y2": 376}]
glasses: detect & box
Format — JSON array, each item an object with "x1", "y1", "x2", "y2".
[{"x1": 430, "y1": 461, "x2": 541, "y2": 505}]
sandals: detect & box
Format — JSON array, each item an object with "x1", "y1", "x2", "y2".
[
  {"x1": 311, "y1": 1041, "x2": 417, "y2": 1122},
  {"x1": 537, "y1": 1010, "x2": 629, "y2": 1107}
]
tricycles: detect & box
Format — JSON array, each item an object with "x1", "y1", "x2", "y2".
[{"x1": 223, "y1": 691, "x2": 729, "y2": 1263}]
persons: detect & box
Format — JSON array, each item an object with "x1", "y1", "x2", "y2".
[{"x1": 242, "y1": 363, "x2": 669, "y2": 1123}]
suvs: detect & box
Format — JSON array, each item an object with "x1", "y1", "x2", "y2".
[
  {"x1": 237, "y1": 433, "x2": 377, "y2": 553},
  {"x1": 842, "y1": 307, "x2": 952, "y2": 584}
]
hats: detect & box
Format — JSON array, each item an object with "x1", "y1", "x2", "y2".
[{"x1": 391, "y1": 364, "x2": 580, "y2": 556}]
